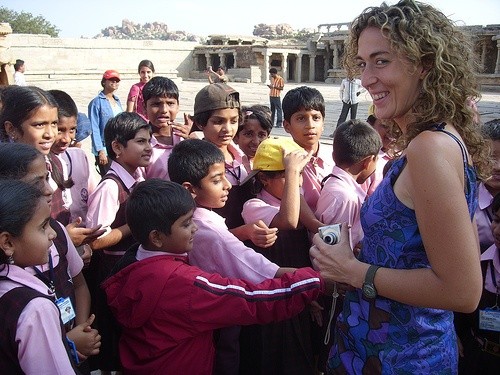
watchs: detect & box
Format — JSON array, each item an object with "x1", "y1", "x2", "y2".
[{"x1": 360, "y1": 264, "x2": 382, "y2": 301}]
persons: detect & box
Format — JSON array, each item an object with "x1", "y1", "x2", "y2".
[
  {"x1": 309, "y1": 0, "x2": 484, "y2": 375},
  {"x1": 127, "y1": 59, "x2": 154, "y2": 123},
  {"x1": 328, "y1": 69, "x2": 366, "y2": 138},
  {"x1": 100, "y1": 178, "x2": 329, "y2": 375},
  {"x1": 88, "y1": 69, "x2": 125, "y2": 178},
  {"x1": 267, "y1": 68, "x2": 284, "y2": 128},
  {"x1": 0, "y1": 176, "x2": 78, "y2": 375},
  {"x1": 0, "y1": 77, "x2": 403, "y2": 362},
  {"x1": 207, "y1": 66, "x2": 228, "y2": 84},
  {"x1": 13, "y1": 59, "x2": 26, "y2": 87},
  {"x1": 457, "y1": 117, "x2": 500, "y2": 375},
  {"x1": 167, "y1": 138, "x2": 303, "y2": 375}
]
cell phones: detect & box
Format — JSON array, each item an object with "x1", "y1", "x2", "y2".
[{"x1": 208, "y1": 66, "x2": 212, "y2": 71}]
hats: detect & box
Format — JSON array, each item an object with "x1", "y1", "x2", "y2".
[
  {"x1": 103, "y1": 70, "x2": 120, "y2": 81},
  {"x1": 239, "y1": 137, "x2": 308, "y2": 186},
  {"x1": 368, "y1": 105, "x2": 375, "y2": 117},
  {"x1": 189, "y1": 83, "x2": 240, "y2": 135}
]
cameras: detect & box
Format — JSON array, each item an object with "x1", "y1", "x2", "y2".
[{"x1": 317, "y1": 223, "x2": 342, "y2": 245}]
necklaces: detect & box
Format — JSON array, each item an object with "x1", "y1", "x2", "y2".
[
  {"x1": 32, "y1": 253, "x2": 76, "y2": 325},
  {"x1": 0, "y1": 274, "x2": 79, "y2": 367},
  {"x1": 225, "y1": 166, "x2": 241, "y2": 185}
]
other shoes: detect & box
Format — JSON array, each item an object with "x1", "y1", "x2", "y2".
[
  {"x1": 329, "y1": 134, "x2": 334, "y2": 137},
  {"x1": 275, "y1": 126, "x2": 281, "y2": 128}
]
